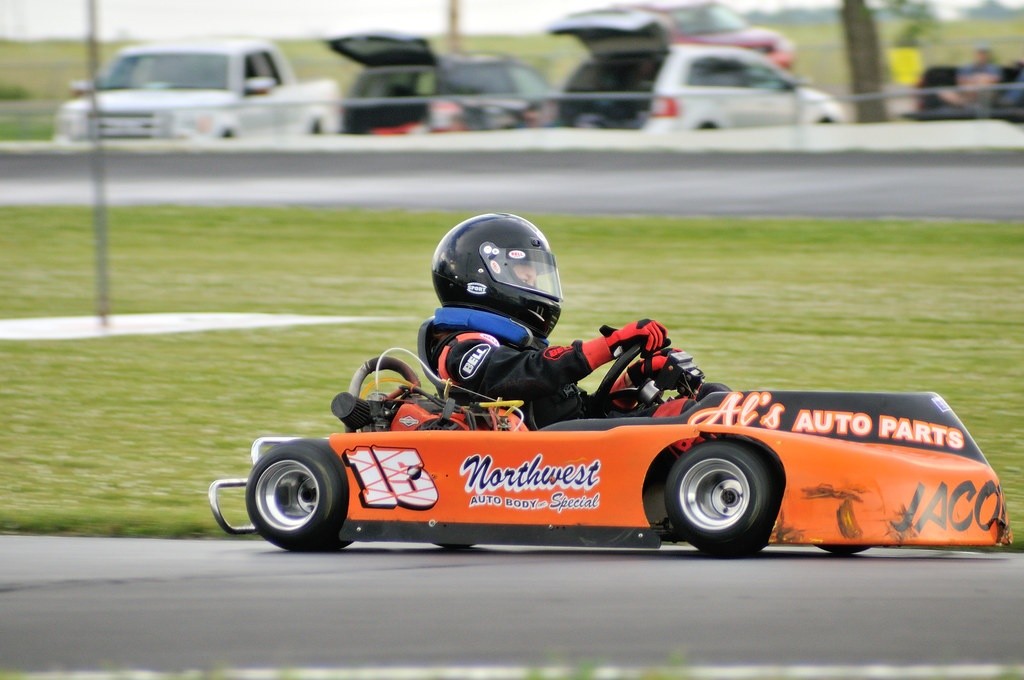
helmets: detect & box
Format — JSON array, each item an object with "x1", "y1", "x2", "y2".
[{"x1": 431, "y1": 213, "x2": 563, "y2": 338}]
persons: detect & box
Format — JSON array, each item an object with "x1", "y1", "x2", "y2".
[
  {"x1": 938, "y1": 41, "x2": 1002, "y2": 119},
  {"x1": 426, "y1": 212, "x2": 733, "y2": 432}
]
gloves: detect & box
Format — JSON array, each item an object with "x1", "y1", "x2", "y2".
[
  {"x1": 581, "y1": 318, "x2": 671, "y2": 372},
  {"x1": 610, "y1": 347, "x2": 685, "y2": 411}
]
